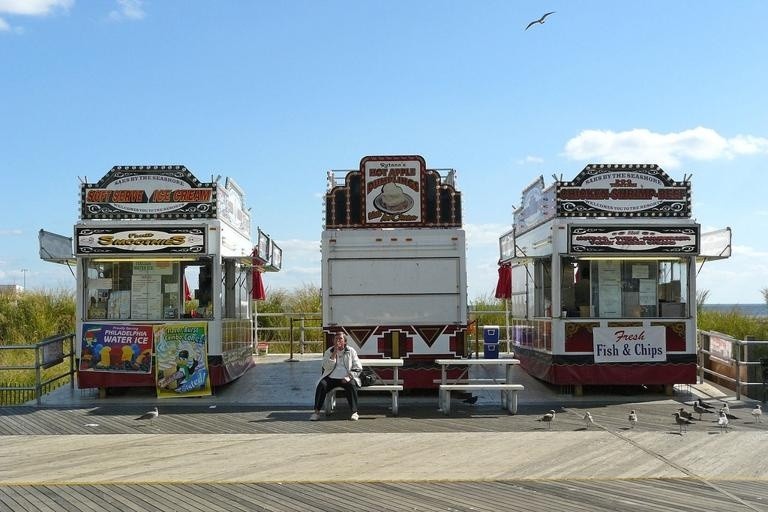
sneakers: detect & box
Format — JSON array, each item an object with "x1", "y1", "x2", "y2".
[
  {"x1": 310, "y1": 413, "x2": 321, "y2": 421},
  {"x1": 350, "y1": 412, "x2": 360, "y2": 420}
]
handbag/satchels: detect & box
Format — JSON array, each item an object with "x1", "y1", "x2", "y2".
[{"x1": 360, "y1": 371, "x2": 376, "y2": 386}]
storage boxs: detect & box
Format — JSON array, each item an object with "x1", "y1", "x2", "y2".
[{"x1": 483, "y1": 324, "x2": 500, "y2": 359}]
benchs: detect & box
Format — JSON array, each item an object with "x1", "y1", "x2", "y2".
[
  {"x1": 432, "y1": 378, "x2": 524, "y2": 415},
  {"x1": 324, "y1": 378, "x2": 404, "y2": 416}
]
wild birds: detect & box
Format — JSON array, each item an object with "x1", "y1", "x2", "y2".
[
  {"x1": 584, "y1": 412, "x2": 593, "y2": 427},
  {"x1": 132, "y1": 407, "x2": 159, "y2": 426},
  {"x1": 629, "y1": 409, "x2": 638, "y2": 429},
  {"x1": 533, "y1": 410, "x2": 556, "y2": 429},
  {"x1": 752, "y1": 404, "x2": 762, "y2": 423},
  {"x1": 524, "y1": 11, "x2": 556, "y2": 31},
  {"x1": 672, "y1": 397, "x2": 740, "y2": 434},
  {"x1": 462, "y1": 396, "x2": 479, "y2": 406}
]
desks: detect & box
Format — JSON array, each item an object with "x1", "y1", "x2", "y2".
[
  {"x1": 435, "y1": 358, "x2": 521, "y2": 415},
  {"x1": 322, "y1": 358, "x2": 405, "y2": 416}
]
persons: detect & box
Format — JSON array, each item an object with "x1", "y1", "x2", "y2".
[{"x1": 309, "y1": 331, "x2": 363, "y2": 422}]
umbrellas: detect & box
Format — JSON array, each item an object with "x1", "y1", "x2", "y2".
[
  {"x1": 185, "y1": 278, "x2": 196, "y2": 316},
  {"x1": 495, "y1": 265, "x2": 511, "y2": 353},
  {"x1": 253, "y1": 250, "x2": 265, "y2": 354}
]
